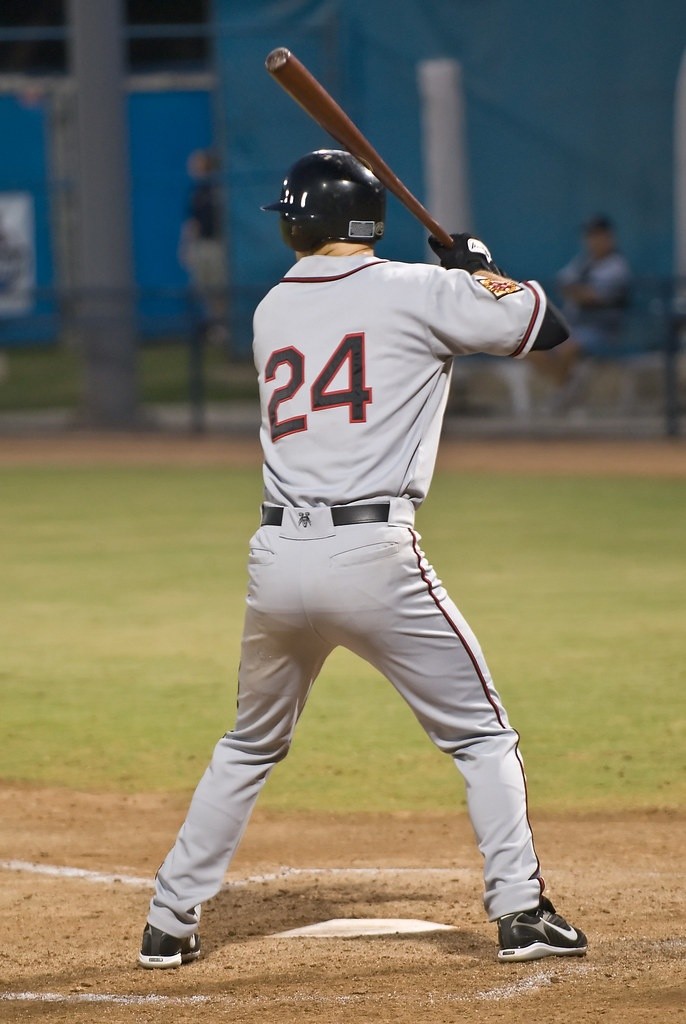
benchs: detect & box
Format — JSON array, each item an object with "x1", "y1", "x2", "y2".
[{"x1": 35, "y1": 274, "x2": 686, "y2": 440}]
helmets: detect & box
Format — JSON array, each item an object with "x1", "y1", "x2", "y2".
[{"x1": 262, "y1": 149, "x2": 387, "y2": 254}]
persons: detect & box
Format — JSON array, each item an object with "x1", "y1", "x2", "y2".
[
  {"x1": 137, "y1": 150, "x2": 586, "y2": 969},
  {"x1": 543, "y1": 209, "x2": 629, "y2": 359},
  {"x1": 178, "y1": 149, "x2": 229, "y2": 346}
]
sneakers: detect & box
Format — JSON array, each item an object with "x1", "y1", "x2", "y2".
[
  {"x1": 138, "y1": 923, "x2": 202, "y2": 968},
  {"x1": 497, "y1": 895, "x2": 588, "y2": 963}
]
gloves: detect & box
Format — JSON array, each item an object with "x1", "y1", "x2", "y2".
[{"x1": 429, "y1": 232, "x2": 509, "y2": 280}]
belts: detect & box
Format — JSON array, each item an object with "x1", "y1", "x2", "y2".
[{"x1": 259, "y1": 503, "x2": 388, "y2": 526}]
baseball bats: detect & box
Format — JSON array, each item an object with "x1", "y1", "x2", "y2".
[{"x1": 257, "y1": 41, "x2": 463, "y2": 255}]
